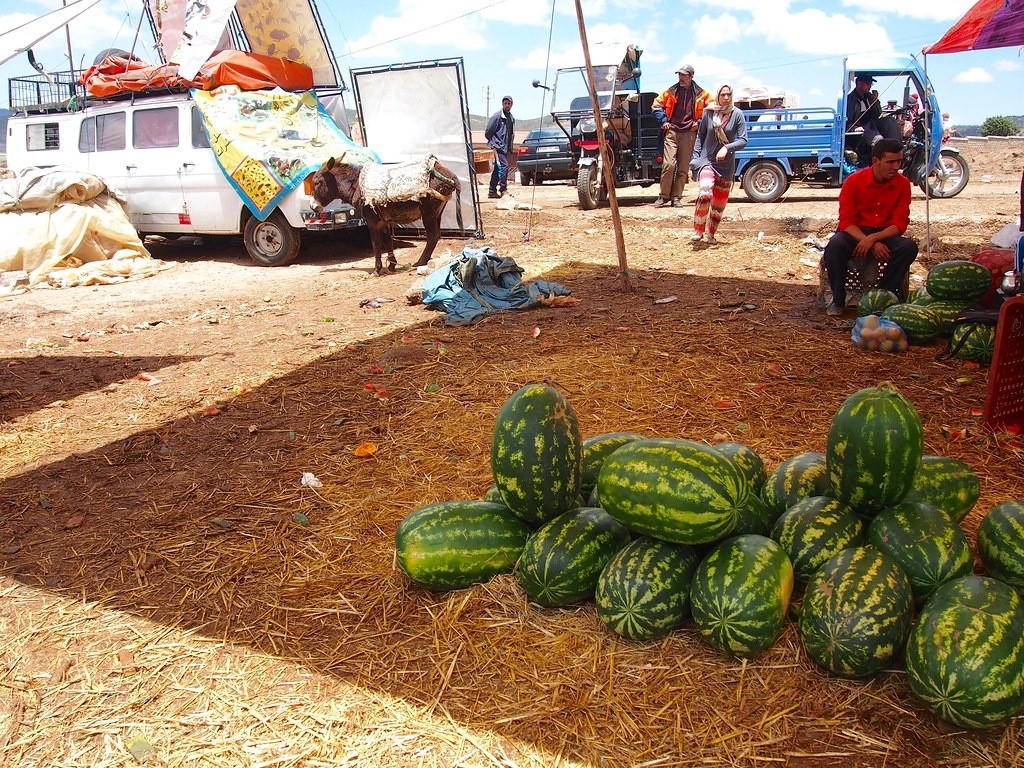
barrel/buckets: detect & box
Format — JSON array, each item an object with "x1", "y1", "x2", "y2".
[{"x1": 515, "y1": 172, "x2": 521, "y2": 183}]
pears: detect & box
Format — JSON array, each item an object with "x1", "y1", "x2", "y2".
[{"x1": 851, "y1": 315, "x2": 909, "y2": 353}]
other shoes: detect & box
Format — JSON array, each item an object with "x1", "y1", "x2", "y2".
[
  {"x1": 828, "y1": 302, "x2": 845, "y2": 315},
  {"x1": 501, "y1": 191, "x2": 514, "y2": 197},
  {"x1": 671, "y1": 200, "x2": 683, "y2": 208},
  {"x1": 655, "y1": 197, "x2": 668, "y2": 205},
  {"x1": 707, "y1": 236, "x2": 717, "y2": 244},
  {"x1": 692, "y1": 232, "x2": 703, "y2": 241},
  {"x1": 488, "y1": 193, "x2": 500, "y2": 198}
]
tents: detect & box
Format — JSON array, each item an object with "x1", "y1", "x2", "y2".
[{"x1": 734, "y1": 84, "x2": 799, "y2": 130}]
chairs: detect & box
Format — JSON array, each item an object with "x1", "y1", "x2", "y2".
[{"x1": 606, "y1": 96, "x2": 632, "y2": 157}]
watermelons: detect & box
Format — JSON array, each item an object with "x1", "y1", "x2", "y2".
[
  {"x1": 856, "y1": 289, "x2": 899, "y2": 317},
  {"x1": 881, "y1": 259, "x2": 997, "y2": 365},
  {"x1": 394, "y1": 383, "x2": 1024, "y2": 731}
]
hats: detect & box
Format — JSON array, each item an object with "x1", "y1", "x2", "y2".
[
  {"x1": 503, "y1": 96, "x2": 513, "y2": 102},
  {"x1": 675, "y1": 65, "x2": 694, "y2": 75},
  {"x1": 857, "y1": 74, "x2": 877, "y2": 82}
]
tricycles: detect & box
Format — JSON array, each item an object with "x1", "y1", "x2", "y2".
[{"x1": 735, "y1": 55, "x2": 971, "y2": 204}]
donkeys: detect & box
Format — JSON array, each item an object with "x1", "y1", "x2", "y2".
[{"x1": 309, "y1": 151, "x2": 465, "y2": 279}]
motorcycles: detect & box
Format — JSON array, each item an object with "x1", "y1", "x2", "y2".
[{"x1": 569, "y1": 91, "x2": 666, "y2": 210}]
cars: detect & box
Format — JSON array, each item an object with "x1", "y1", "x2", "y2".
[{"x1": 515, "y1": 126, "x2": 578, "y2": 186}]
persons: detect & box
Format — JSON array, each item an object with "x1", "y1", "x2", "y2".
[
  {"x1": 752, "y1": 100, "x2": 791, "y2": 129},
  {"x1": 485, "y1": 96, "x2": 516, "y2": 200},
  {"x1": 823, "y1": 138, "x2": 918, "y2": 315},
  {"x1": 689, "y1": 85, "x2": 748, "y2": 245},
  {"x1": 847, "y1": 74, "x2": 904, "y2": 156},
  {"x1": 651, "y1": 64, "x2": 714, "y2": 208}
]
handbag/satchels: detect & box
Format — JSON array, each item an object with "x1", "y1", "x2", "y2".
[
  {"x1": 851, "y1": 315, "x2": 908, "y2": 352},
  {"x1": 954, "y1": 307, "x2": 998, "y2": 326}
]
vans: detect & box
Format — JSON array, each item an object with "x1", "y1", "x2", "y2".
[{"x1": 6, "y1": 88, "x2": 374, "y2": 267}]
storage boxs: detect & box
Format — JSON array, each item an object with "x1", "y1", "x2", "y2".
[
  {"x1": 983, "y1": 295, "x2": 1024, "y2": 453},
  {"x1": 817, "y1": 257, "x2": 909, "y2": 308}
]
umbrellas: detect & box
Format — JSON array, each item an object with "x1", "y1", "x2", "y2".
[{"x1": 923, "y1": 0, "x2": 1024, "y2": 55}]
